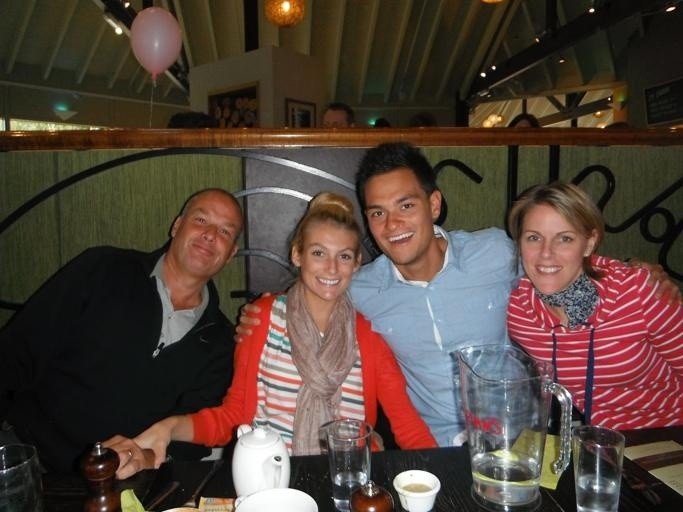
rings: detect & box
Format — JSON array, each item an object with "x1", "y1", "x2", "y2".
[{"x1": 127, "y1": 448, "x2": 133, "y2": 460}]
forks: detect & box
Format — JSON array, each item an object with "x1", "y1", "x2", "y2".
[{"x1": 581, "y1": 437, "x2": 662, "y2": 491}]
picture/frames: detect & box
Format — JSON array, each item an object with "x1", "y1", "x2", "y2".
[
  {"x1": 284, "y1": 98, "x2": 317, "y2": 128},
  {"x1": 207, "y1": 80, "x2": 260, "y2": 128}
]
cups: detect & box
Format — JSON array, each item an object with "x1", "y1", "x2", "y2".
[
  {"x1": 570, "y1": 426, "x2": 626, "y2": 511},
  {"x1": 392, "y1": 468, "x2": 443, "y2": 511},
  {"x1": 326, "y1": 419, "x2": 372, "y2": 512},
  {"x1": 0, "y1": 442, "x2": 43, "y2": 512}
]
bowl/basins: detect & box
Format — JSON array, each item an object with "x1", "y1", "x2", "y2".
[{"x1": 234, "y1": 488, "x2": 319, "y2": 511}]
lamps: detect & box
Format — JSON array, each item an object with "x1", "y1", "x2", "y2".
[{"x1": 264, "y1": 0, "x2": 306, "y2": 30}]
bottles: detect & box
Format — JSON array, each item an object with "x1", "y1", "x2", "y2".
[
  {"x1": 347, "y1": 479, "x2": 394, "y2": 512},
  {"x1": 78, "y1": 442, "x2": 121, "y2": 512}
]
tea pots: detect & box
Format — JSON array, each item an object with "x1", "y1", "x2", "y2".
[
  {"x1": 454, "y1": 344, "x2": 573, "y2": 511},
  {"x1": 230, "y1": 422, "x2": 291, "y2": 497}
]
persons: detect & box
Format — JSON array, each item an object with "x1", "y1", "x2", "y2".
[
  {"x1": 318, "y1": 104, "x2": 355, "y2": 131},
  {"x1": 507, "y1": 113, "x2": 539, "y2": 128},
  {"x1": 233, "y1": 142, "x2": 680, "y2": 450},
  {"x1": 163, "y1": 113, "x2": 220, "y2": 129},
  {"x1": 602, "y1": 121, "x2": 636, "y2": 129},
  {"x1": 1, "y1": 189, "x2": 249, "y2": 480},
  {"x1": 504, "y1": 181, "x2": 682, "y2": 430},
  {"x1": 129, "y1": 192, "x2": 440, "y2": 472},
  {"x1": 409, "y1": 112, "x2": 436, "y2": 126},
  {"x1": 373, "y1": 118, "x2": 390, "y2": 127}
]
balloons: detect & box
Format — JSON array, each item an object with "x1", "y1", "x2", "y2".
[{"x1": 129, "y1": 6, "x2": 185, "y2": 82}]
